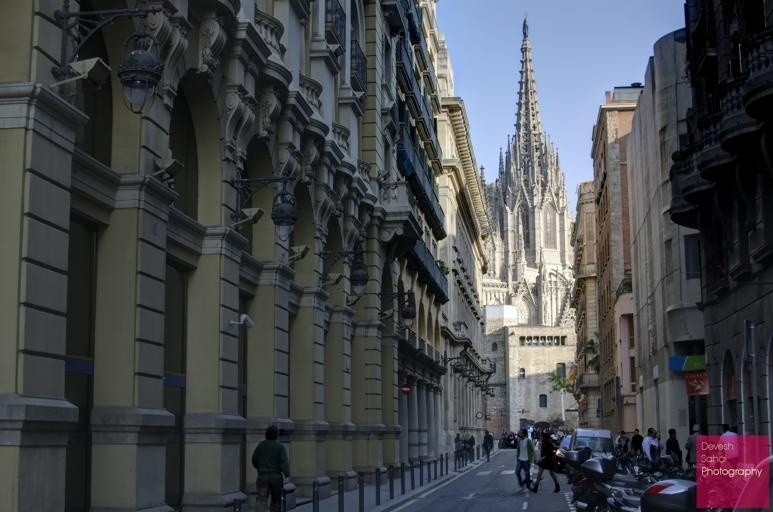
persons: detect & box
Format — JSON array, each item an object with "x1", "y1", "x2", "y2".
[
  {"x1": 252, "y1": 425, "x2": 291, "y2": 511},
  {"x1": 481, "y1": 429, "x2": 494, "y2": 462},
  {"x1": 641, "y1": 427, "x2": 663, "y2": 462},
  {"x1": 716, "y1": 424, "x2": 738, "y2": 468},
  {"x1": 498, "y1": 425, "x2": 570, "y2": 493},
  {"x1": 666, "y1": 428, "x2": 682, "y2": 466},
  {"x1": 613, "y1": 432, "x2": 621, "y2": 450},
  {"x1": 685, "y1": 423, "x2": 705, "y2": 474},
  {"x1": 630, "y1": 429, "x2": 643, "y2": 457},
  {"x1": 453, "y1": 432, "x2": 475, "y2": 466},
  {"x1": 616, "y1": 430, "x2": 629, "y2": 455}
]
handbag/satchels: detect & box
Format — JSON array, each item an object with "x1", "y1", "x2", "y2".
[{"x1": 552, "y1": 452, "x2": 556, "y2": 467}]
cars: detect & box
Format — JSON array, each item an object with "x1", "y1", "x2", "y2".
[{"x1": 550, "y1": 425, "x2": 616, "y2": 473}]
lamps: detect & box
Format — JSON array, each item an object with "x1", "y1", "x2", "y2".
[
  {"x1": 441, "y1": 356, "x2": 487, "y2": 392},
  {"x1": 317, "y1": 245, "x2": 417, "y2": 328},
  {"x1": 221, "y1": 172, "x2": 301, "y2": 243},
  {"x1": 50, "y1": 2, "x2": 186, "y2": 116}
]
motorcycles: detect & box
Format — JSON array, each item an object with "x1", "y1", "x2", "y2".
[{"x1": 561, "y1": 442, "x2": 747, "y2": 512}]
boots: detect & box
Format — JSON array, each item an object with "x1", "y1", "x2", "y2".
[
  {"x1": 553, "y1": 482, "x2": 560, "y2": 493},
  {"x1": 529, "y1": 482, "x2": 539, "y2": 493}
]
